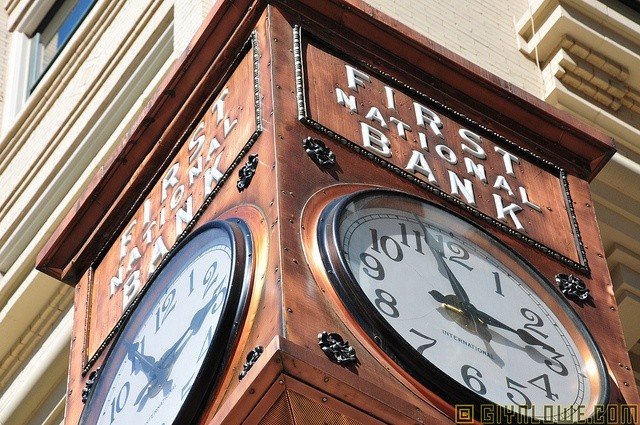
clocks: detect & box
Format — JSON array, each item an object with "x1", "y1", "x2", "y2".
[
  {"x1": 318, "y1": 185, "x2": 610, "y2": 424},
  {"x1": 78, "y1": 217, "x2": 254, "y2": 425}
]
